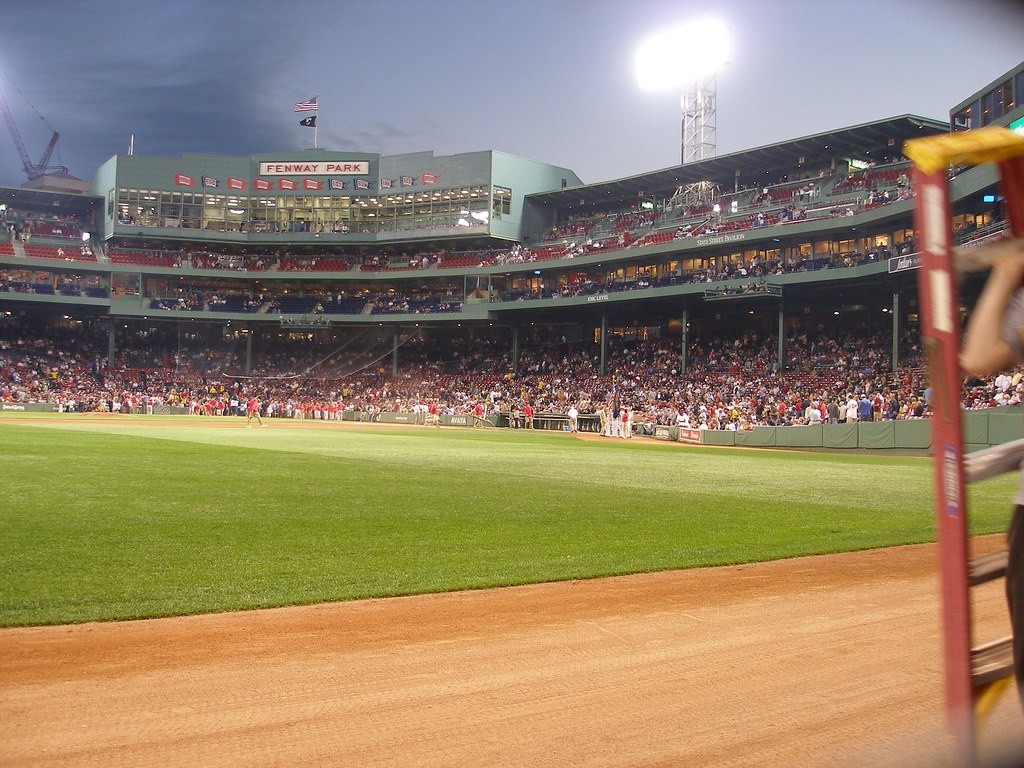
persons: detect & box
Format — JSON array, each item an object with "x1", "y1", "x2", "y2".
[
  {"x1": 1, "y1": 156, "x2": 1024, "y2": 439},
  {"x1": 962, "y1": 243, "x2": 1024, "y2": 709}
]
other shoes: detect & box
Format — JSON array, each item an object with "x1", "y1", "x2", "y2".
[
  {"x1": 575, "y1": 430, "x2": 577, "y2": 433},
  {"x1": 571, "y1": 431, "x2": 573, "y2": 433},
  {"x1": 248, "y1": 426, "x2": 252, "y2": 428},
  {"x1": 261, "y1": 425, "x2": 267, "y2": 427},
  {"x1": 479, "y1": 426, "x2": 484, "y2": 428},
  {"x1": 473, "y1": 425, "x2": 477, "y2": 427}
]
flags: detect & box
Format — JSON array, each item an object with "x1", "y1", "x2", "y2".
[
  {"x1": 300, "y1": 116, "x2": 316, "y2": 127},
  {"x1": 295, "y1": 95, "x2": 317, "y2": 112}
]
